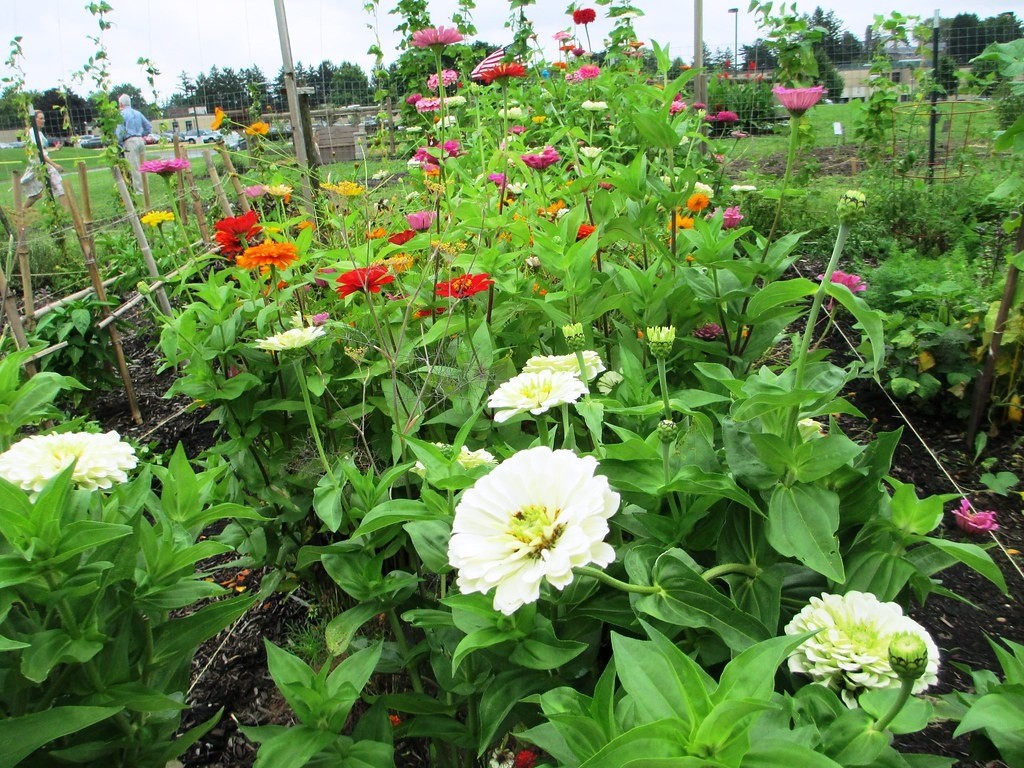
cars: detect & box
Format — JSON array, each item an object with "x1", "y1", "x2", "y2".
[
  {"x1": 0, "y1": 135, "x2": 112, "y2": 149},
  {"x1": 144, "y1": 122, "x2": 294, "y2": 152},
  {"x1": 311, "y1": 114, "x2": 378, "y2": 129}
]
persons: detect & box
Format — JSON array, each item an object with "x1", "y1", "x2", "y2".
[
  {"x1": 116, "y1": 94, "x2": 152, "y2": 196},
  {"x1": 20, "y1": 110, "x2": 68, "y2": 209}
]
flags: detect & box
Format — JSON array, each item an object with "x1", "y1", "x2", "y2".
[{"x1": 471, "y1": 44, "x2": 513, "y2": 80}]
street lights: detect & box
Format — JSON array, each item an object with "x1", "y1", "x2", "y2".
[{"x1": 727, "y1": 9, "x2": 738, "y2": 71}]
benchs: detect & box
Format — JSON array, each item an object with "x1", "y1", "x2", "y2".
[{"x1": 315, "y1": 127, "x2": 356, "y2": 164}]
